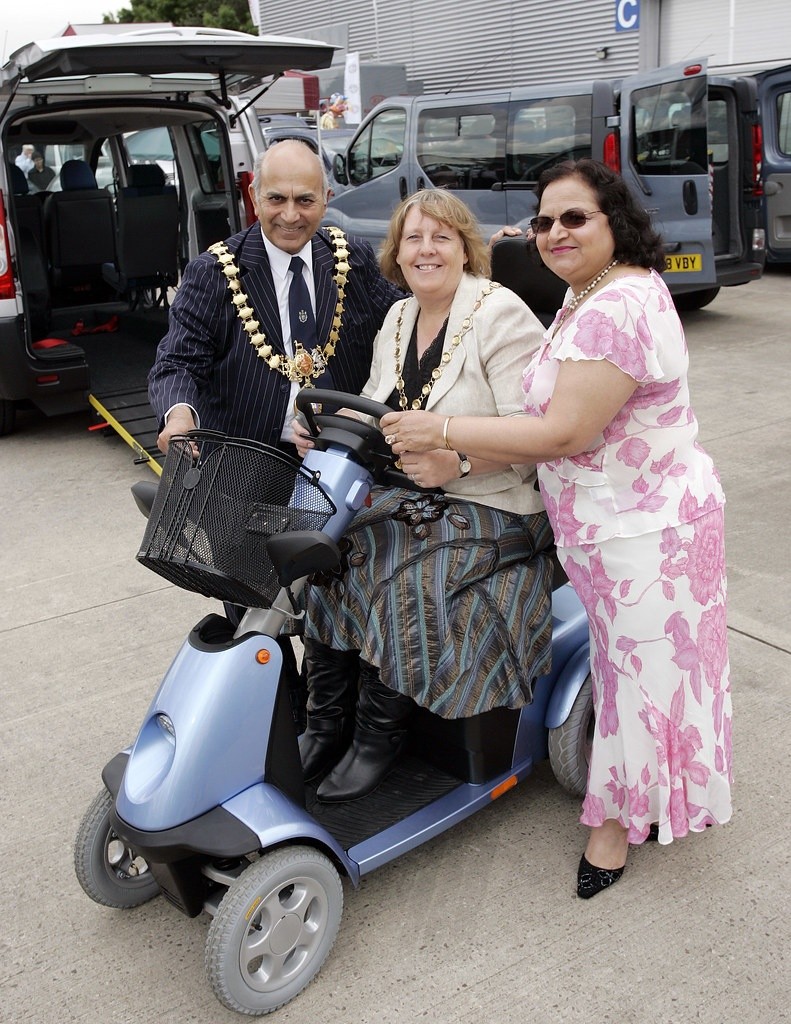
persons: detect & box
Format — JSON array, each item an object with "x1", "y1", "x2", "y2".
[
  {"x1": 320, "y1": 93, "x2": 349, "y2": 130},
  {"x1": 15, "y1": 144, "x2": 35, "y2": 179},
  {"x1": 290, "y1": 189, "x2": 551, "y2": 802},
  {"x1": 28, "y1": 151, "x2": 55, "y2": 190},
  {"x1": 378, "y1": 158, "x2": 734, "y2": 899},
  {"x1": 148, "y1": 139, "x2": 412, "y2": 626}
]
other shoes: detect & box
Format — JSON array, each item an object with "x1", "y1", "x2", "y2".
[
  {"x1": 646, "y1": 821, "x2": 670, "y2": 843},
  {"x1": 577, "y1": 853, "x2": 626, "y2": 900}
]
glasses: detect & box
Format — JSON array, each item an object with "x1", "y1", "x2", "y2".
[{"x1": 528, "y1": 209, "x2": 606, "y2": 235}]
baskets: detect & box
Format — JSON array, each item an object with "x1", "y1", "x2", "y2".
[{"x1": 135, "y1": 429, "x2": 337, "y2": 609}]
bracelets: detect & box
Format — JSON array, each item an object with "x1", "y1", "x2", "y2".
[{"x1": 443, "y1": 416, "x2": 453, "y2": 450}]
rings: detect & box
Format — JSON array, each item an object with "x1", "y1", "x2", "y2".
[
  {"x1": 385, "y1": 435, "x2": 394, "y2": 445},
  {"x1": 412, "y1": 474, "x2": 415, "y2": 479},
  {"x1": 418, "y1": 482, "x2": 421, "y2": 486}
]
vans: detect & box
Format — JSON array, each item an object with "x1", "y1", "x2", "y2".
[
  {"x1": 259, "y1": 56, "x2": 791, "y2": 312},
  {"x1": 0, "y1": 25, "x2": 345, "y2": 441}
]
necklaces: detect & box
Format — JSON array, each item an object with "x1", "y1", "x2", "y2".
[
  {"x1": 554, "y1": 260, "x2": 618, "y2": 333},
  {"x1": 207, "y1": 227, "x2": 352, "y2": 415},
  {"x1": 394, "y1": 283, "x2": 501, "y2": 469}
]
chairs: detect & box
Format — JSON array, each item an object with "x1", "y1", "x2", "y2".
[
  {"x1": 7, "y1": 160, "x2": 180, "y2": 337},
  {"x1": 487, "y1": 235, "x2": 568, "y2": 591}
]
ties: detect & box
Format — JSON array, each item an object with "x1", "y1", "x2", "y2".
[{"x1": 288, "y1": 256, "x2": 340, "y2": 414}]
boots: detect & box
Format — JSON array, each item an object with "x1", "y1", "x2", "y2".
[
  {"x1": 294, "y1": 636, "x2": 354, "y2": 784},
  {"x1": 316, "y1": 659, "x2": 407, "y2": 805}
]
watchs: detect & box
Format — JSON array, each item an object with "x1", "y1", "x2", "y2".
[{"x1": 458, "y1": 454, "x2": 471, "y2": 478}]
